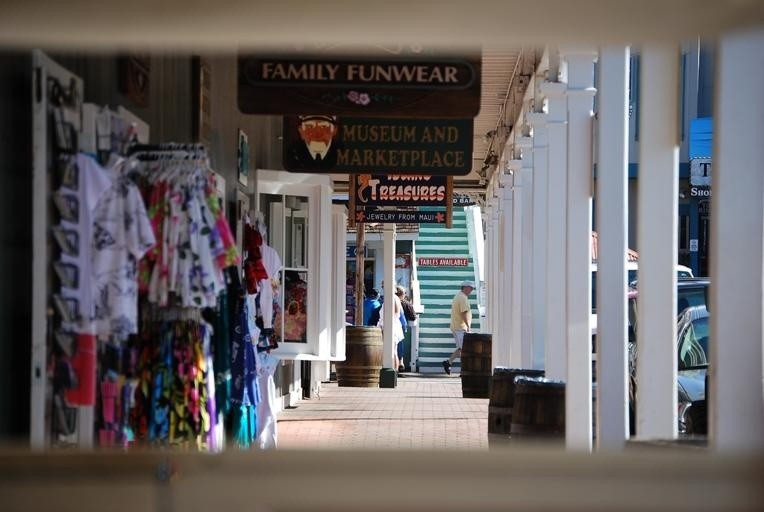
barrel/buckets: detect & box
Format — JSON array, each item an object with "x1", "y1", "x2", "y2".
[
  {"x1": 335, "y1": 325, "x2": 384, "y2": 387},
  {"x1": 509, "y1": 380, "x2": 565, "y2": 437},
  {"x1": 487, "y1": 369, "x2": 546, "y2": 435},
  {"x1": 460, "y1": 332, "x2": 493, "y2": 400}
]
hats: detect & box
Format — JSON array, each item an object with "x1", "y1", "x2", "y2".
[{"x1": 462, "y1": 280, "x2": 475, "y2": 289}]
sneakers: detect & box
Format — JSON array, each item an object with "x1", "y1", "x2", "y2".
[
  {"x1": 399, "y1": 364, "x2": 406, "y2": 373},
  {"x1": 443, "y1": 359, "x2": 451, "y2": 375}
]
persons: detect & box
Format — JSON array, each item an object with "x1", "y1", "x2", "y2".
[
  {"x1": 362, "y1": 280, "x2": 417, "y2": 375},
  {"x1": 442, "y1": 280, "x2": 473, "y2": 375}
]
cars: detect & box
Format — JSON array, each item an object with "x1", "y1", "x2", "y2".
[{"x1": 592, "y1": 260, "x2": 707, "y2": 441}]
